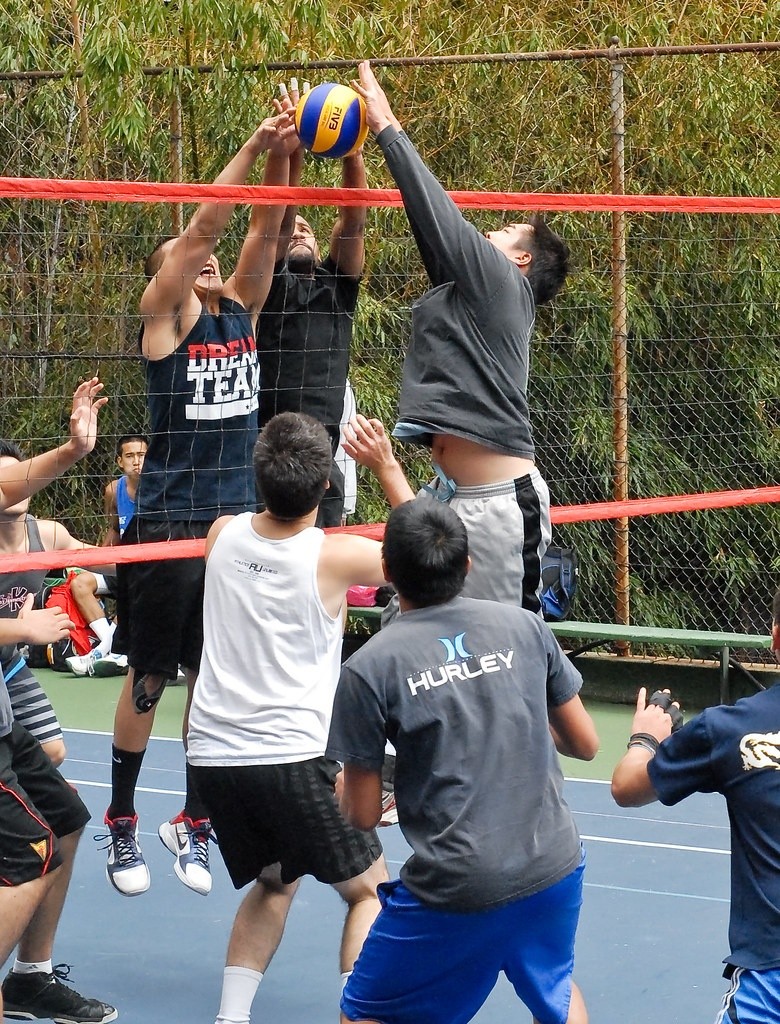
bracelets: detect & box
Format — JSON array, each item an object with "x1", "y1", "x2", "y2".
[{"x1": 628, "y1": 736, "x2": 656, "y2": 752}]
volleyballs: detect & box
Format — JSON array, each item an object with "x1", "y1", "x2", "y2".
[{"x1": 293, "y1": 83, "x2": 371, "y2": 159}]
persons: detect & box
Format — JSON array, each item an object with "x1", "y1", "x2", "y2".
[
  {"x1": 1, "y1": 375, "x2": 152, "y2": 1024},
  {"x1": 326, "y1": 497, "x2": 598, "y2": 1024},
  {"x1": 612, "y1": 588, "x2": 779, "y2": 1023},
  {"x1": 95, "y1": 60, "x2": 570, "y2": 898},
  {"x1": 187, "y1": 411, "x2": 417, "y2": 1023}
]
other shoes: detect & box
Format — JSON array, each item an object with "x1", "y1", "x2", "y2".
[{"x1": 377, "y1": 789, "x2": 399, "y2": 827}]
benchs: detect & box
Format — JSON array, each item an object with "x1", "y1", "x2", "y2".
[{"x1": 542, "y1": 620, "x2": 775, "y2": 706}]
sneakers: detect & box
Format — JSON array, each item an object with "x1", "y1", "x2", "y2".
[
  {"x1": 1, "y1": 963, "x2": 118, "y2": 1024},
  {"x1": 93, "y1": 653, "x2": 129, "y2": 678},
  {"x1": 65, "y1": 653, "x2": 96, "y2": 677},
  {"x1": 158, "y1": 809, "x2": 219, "y2": 896},
  {"x1": 164, "y1": 663, "x2": 185, "y2": 687},
  {"x1": 93, "y1": 804, "x2": 150, "y2": 897}
]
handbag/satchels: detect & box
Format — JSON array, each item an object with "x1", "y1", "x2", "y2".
[{"x1": 346, "y1": 584, "x2": 398, "y2": 607}]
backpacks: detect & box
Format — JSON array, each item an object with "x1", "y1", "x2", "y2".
[
  {"x1": 17, "y1": 567, "x2": 112, "y2": 672},
  {"x1": 540, "y1": 544, "x2": 581, "y2": 622}
]
